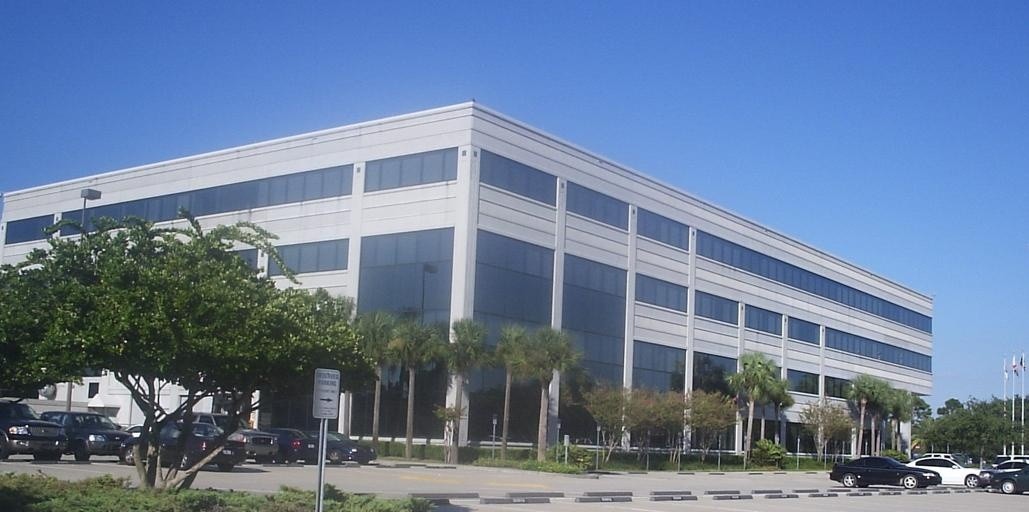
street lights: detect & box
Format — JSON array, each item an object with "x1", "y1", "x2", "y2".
[
  {"x1": 555, "y1": 418, "x2": 563, "y2": 465},
  {"x1": 490, "y1": 412, "x2": 498, "y2": 460},
  {"x1": 595, "y1": 423, "x2": 603, "y2": 471},
  {"x1": 62, "y1": 188, "x2": 103, "y2": 412}
]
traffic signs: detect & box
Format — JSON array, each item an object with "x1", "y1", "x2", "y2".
[{"x1": 313, "y1": 368, "x2": 340, "y2": 420}]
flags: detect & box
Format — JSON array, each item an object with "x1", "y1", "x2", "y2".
[
  {"x1": 1019, "y1": 355, "x2": 1027, "y2": 373},
  {"x1": 1013, "y1": 356, "x2": 1019, "y2": 378},
  {"x1": 1004, "y1": 362, "x2": 1009, "y2": 380}
]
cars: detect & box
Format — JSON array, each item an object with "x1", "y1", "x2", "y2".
[
  {"x1": 119, "y1": 421, "x2": 247, "y2": 470},
  {"x1": 264, "y1": 427, "x2": 320, "y2": 465},
  {"x1": 317, "y1": 429, "x2": 376, "y2": 466},
  {"x1": 829, "y1": 453, "x2": 1028, "y2": 495},
  {"x1": 124, "y1": 423, "x2": 151, "y2": 439}
]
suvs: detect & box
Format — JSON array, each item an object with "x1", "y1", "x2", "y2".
[
  {"x1": 38, "y1": 410, "x2": 133, "y2": 463},
  {"x1": 172, "y1": 412, "x2": 281, "y2": 465},
  {"x1": 0, "y1": 399, "x2": 70, "y2": 463}
]
